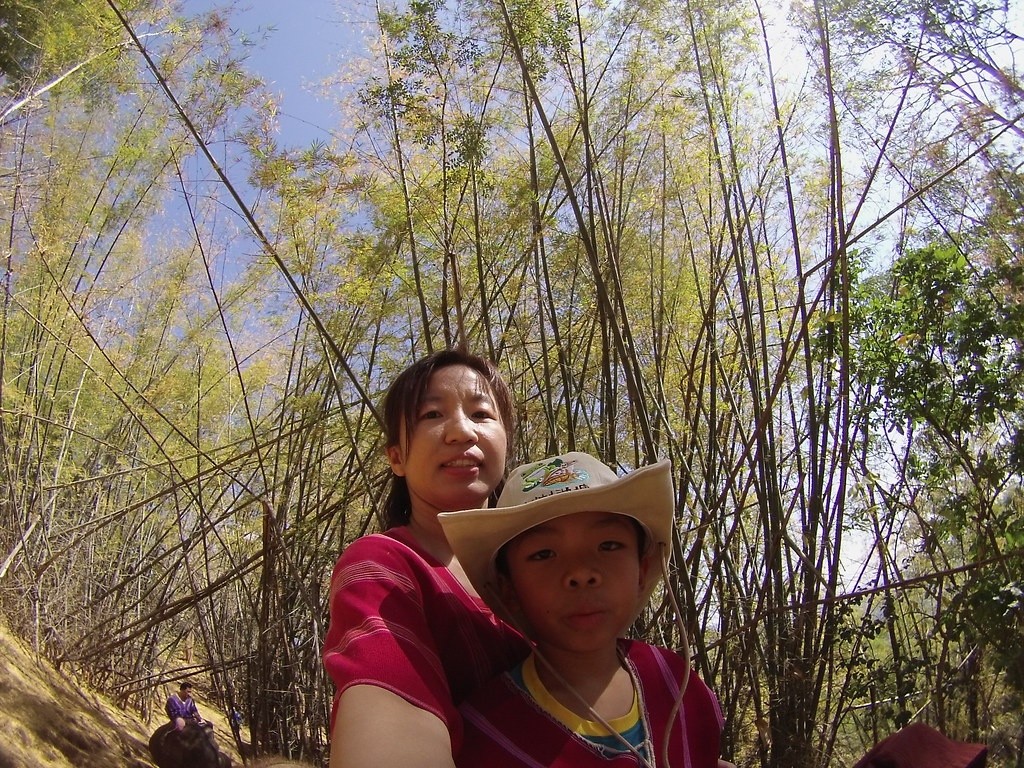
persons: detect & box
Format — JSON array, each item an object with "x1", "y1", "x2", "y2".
[
  {"x1": 323, "y1": 349, "x2": 733, "y2": 768},
  {"x1": 437, "y1": 452, "x2": 724, "y2": 768},
  {"x1": 165, "y1": 682, "x2": 207, "y2": 730},
  {"x1": 230, "y1": 707, "x2": 242, "y2": 742}
]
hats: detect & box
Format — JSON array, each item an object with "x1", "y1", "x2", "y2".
[
  {"x1": 435, "y1": 452, "x2": 678, "y2": 640},
  {"x1": 853, "y1": 724, "x2": 988, "y2": 768}
]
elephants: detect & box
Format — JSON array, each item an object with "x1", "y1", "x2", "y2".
[{"x1": 148, "y1": 721, "x2": 232, "y2": 768}]
